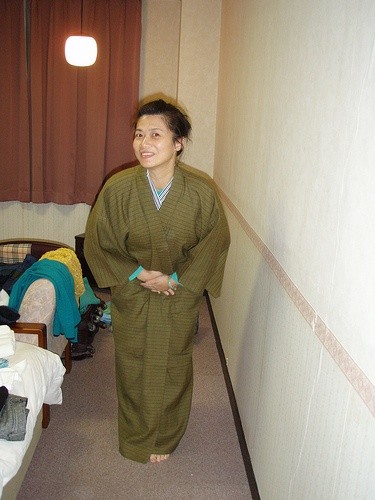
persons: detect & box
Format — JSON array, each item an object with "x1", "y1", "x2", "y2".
[{"x1": 83, "y1": 99, "x2": 231, "y2": 464}]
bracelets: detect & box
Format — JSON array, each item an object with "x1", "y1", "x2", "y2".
[{"x1": 167, "y1": 276, "x2": 177, "y2": 291}]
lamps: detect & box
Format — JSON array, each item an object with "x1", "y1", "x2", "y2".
[{"x1": 64, "y1": 0, "x2": 97, "y2": 67}]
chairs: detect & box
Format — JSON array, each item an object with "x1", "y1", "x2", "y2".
[{"x1": 0, "y1": 239, "x2": 75, "y2": 429}]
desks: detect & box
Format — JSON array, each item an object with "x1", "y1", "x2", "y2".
[{"x1": 75, "y1": 232, "x2": 111, "y2": 294}]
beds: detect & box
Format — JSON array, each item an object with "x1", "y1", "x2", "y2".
[{"x1": 0, "y1": 343, "x2": 66, "y2": 500}]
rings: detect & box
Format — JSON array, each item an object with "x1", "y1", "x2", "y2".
[{"x1": 158, "y1": 290, "x2": 161, "y2": 295}]
